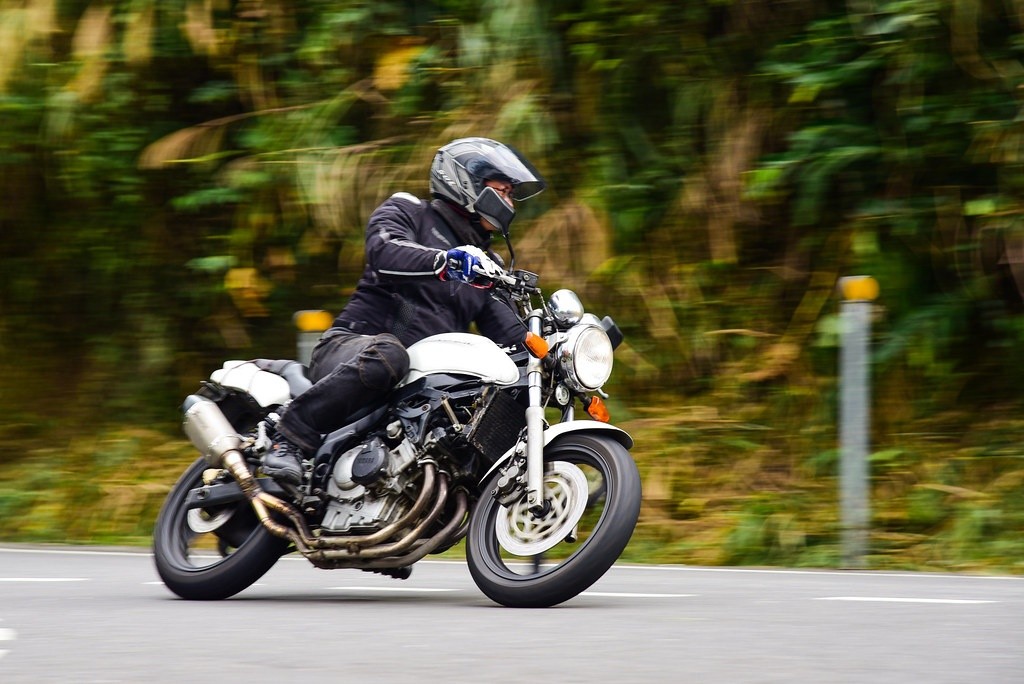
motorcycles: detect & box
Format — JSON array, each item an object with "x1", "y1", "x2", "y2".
[{"x1": 151, "y1": 188, "x2": 642, "y2": 608}]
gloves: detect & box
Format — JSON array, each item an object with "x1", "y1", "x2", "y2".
[{"x1": 433, "y1": 244, "x2": 501, "y2": 290}]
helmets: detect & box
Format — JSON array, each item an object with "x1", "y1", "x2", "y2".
[{"x1": 428, "y1": 138, "x2": 523, "y2": 214}]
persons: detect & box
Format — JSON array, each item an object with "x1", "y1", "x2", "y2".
[{"x1": 257, "y1": 136, "x2": 544, "y2": 482}]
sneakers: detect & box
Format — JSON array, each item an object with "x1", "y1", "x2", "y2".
[{"x1": 263, "y1": 435, "x2": 305, "y2": 485}]
